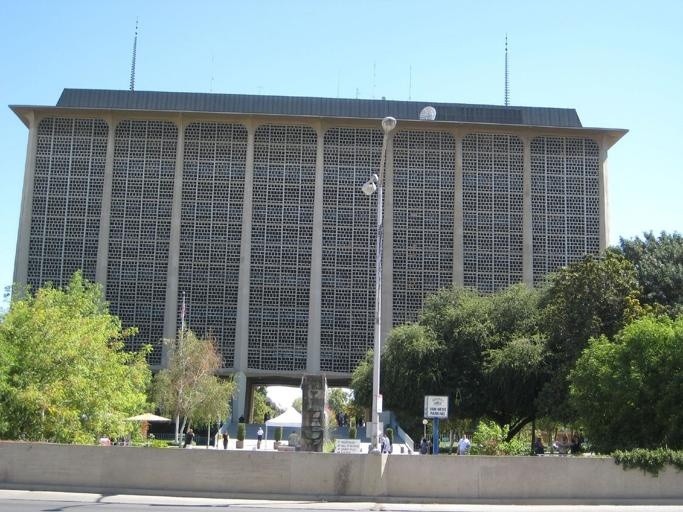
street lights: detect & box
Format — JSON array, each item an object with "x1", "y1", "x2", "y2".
[
  {"x1": 422, "y1": 419, "x2": 427, "y2": 438},
  {"x1": 360, "y1": 115, "x2": 398, "y2": 453}
]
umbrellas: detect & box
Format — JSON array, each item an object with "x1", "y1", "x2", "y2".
[{"x1": 122, "y1": 412, "x2": 171, "y2": 422}]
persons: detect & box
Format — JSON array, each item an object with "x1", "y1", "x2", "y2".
[
  {"x1": 288, "y1": 431, "x2": 298, "y2": 446},
  {"x1": 537, "y1": 429, "x2": 584, "y2": 454},
  {"x1": 264, "y1": 412, "x2": 270, "y2": 422},
  {"x1": 183, "y1": 428, "x2": 195, "y2": 448},
  {"x1": 420, "y1": 433, "x2": 440, "y2": 453},
  {"x1": 336, "y1": 410, "x2": 348, "y2": 427},
  {"x1": 256, "y1": 426, "x2": 263, "y2": 442},
  {"x1": 223, "y1": 430, "x2": 229, "y2": 449},
  {"x1": 457, "y1": 434, "x2": 470, "y2": 454},
  {"x1": 239, "y1": 414, "x2": 245, "y2": 423}
]
